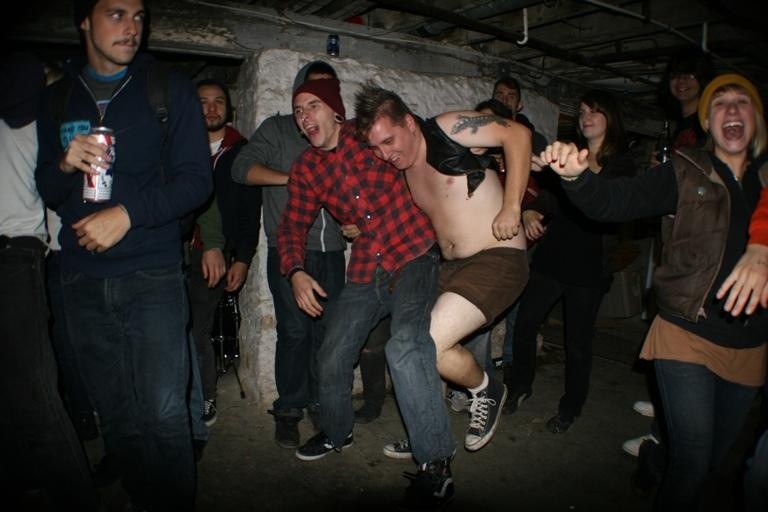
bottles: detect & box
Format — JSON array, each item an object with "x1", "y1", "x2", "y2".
[{"x1": 655, "y1": 117, "x2": 672, "y2": 167}]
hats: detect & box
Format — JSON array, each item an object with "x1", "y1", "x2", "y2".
[
  {"x1": 698, "y1": 74, "x2": 763, "y2": 133},
  {"x1": 291, "y1": 78, "x2": 345, "y2": 118}
]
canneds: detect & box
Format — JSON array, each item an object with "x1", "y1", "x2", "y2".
[
  {"x1": 327, "y1": 34, "x2": 339, "y2": 57},
  {"x1": 83, "y1": 125, "x2": 117, "y2": 204}
]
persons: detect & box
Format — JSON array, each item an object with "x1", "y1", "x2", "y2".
[
  {"x1": 2, "y1": 2, "x2": 264, "y2": 511},
  {"x1": 232, "y1": 52, "x2": 767, "y2": 512}
]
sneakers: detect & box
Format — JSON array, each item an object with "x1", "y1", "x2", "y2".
[
  {"x1": 274, "y1": 417, "x2": 299, "y2": 449},
  {"x1": 634, "y1": 400, "x2": 656, "y2": 417},
  {"x1": 404, "y1": 458, "x2": 455, "y2": 501},
  {"x1": 353, "y1": 406, "x2": 381, "y2": 423},
  {"x1": 622, "y1": 433, "x2": 659, "y2": 456},
  {"x1": 295, "y1": 430, "x2": 353, "y2": 460},
  {"x1": 547, "y1": 412, "x2": 577, "y2": 433},
  {"x1": 383, "y1": 438, "x2": 412, "y2": 458},
  {"x1": 450, "y1": 373, "x2": 533, "y2": 451},
  {"x1": 202, "y1": 399, "x2": 217, "y2": 426}
]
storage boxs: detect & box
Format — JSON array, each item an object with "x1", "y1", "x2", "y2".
[{"x1": 598, "y1": 269, "x2": 642, "y2": 318}]
